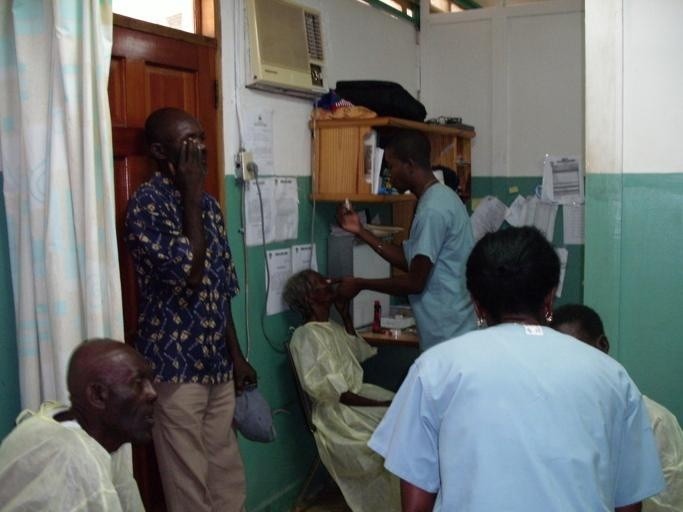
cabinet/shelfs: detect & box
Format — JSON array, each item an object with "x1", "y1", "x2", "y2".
[{"x1": 309, "y1": 118, "x2": 476, "y2": 349}]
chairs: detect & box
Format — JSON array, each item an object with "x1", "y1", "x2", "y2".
[{"x1": 283, "y1": 326, "x2": 326, "y2": 509}]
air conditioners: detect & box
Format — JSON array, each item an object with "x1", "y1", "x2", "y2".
[{"x1": 245, "y1": 0, "x2": 329, "y2": 102}]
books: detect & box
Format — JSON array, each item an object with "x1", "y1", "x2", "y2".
[{"x1": 363, "y1": 129, "x2": 377, "y2": 194}]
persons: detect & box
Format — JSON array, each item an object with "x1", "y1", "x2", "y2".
[
  {"x1": 367, "y1": 223, "x2": 666, "y2": 512},
  {"x1": 545, "y1": 304, "x2": 683, "y2": 511},
  {"x1": 325, "y1": 127, "x2": 481, "y2": 356},
  {"x1": 123, "y1": 107, "x2": 258, "y2": 512},
  {"x1": 283, "y1": 270, "x2": 396, "y2": 439},
  {"x1": 0, "y1": 338, "x2": 158, "y2": 512}
]
